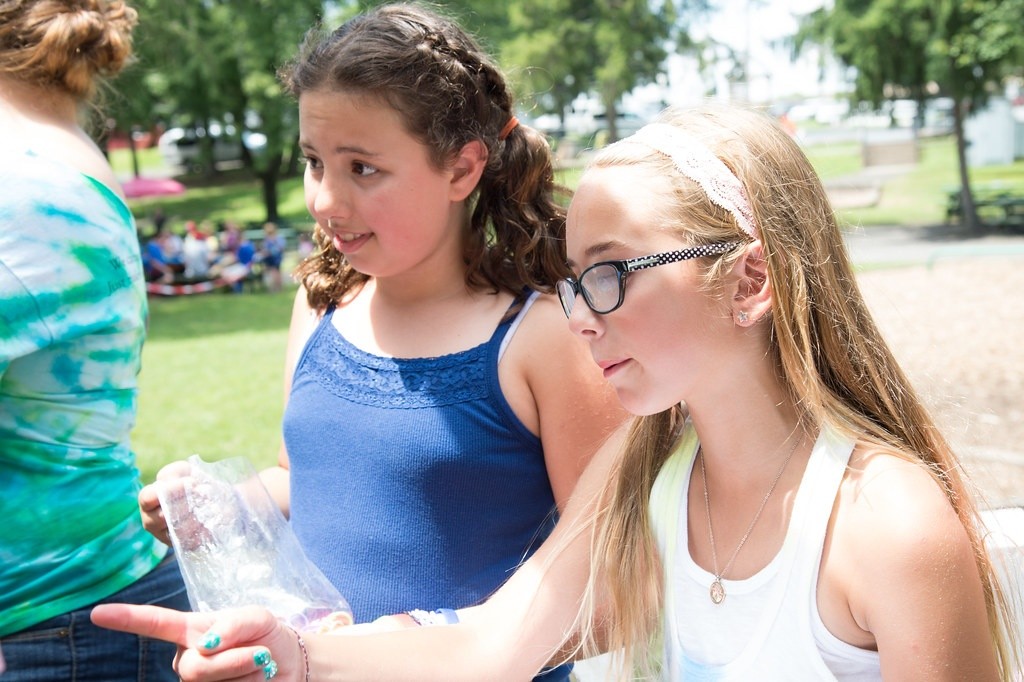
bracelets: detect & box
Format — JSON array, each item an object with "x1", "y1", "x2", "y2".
[
  {"x1": 286, "y1": 625, "x2": 310, "y2": 682},
  {"x1": 406, "y1": 607, "x2": 460, "y2": 628}
]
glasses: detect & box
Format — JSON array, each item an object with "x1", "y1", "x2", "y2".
[{"x1": 555, "y1": 241, "x2": 744, "y2": 319}]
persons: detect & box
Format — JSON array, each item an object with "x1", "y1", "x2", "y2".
[
  {"x1": 91, "y1": 100, "x2": 1024, "y2": 682},
  {"x1": 0, "y1": 0, "x2": 193, "y2": 682},
  {"x1": 138, "y1": 216, "x2": 310, "y2": 293},
  {"x1": 137, "y1": 6, "x2": 633, "y2": 682}
]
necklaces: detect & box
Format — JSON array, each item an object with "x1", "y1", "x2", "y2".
[{"x1": 699, "y1": 424, "x2": 807, "y2": 606}]
